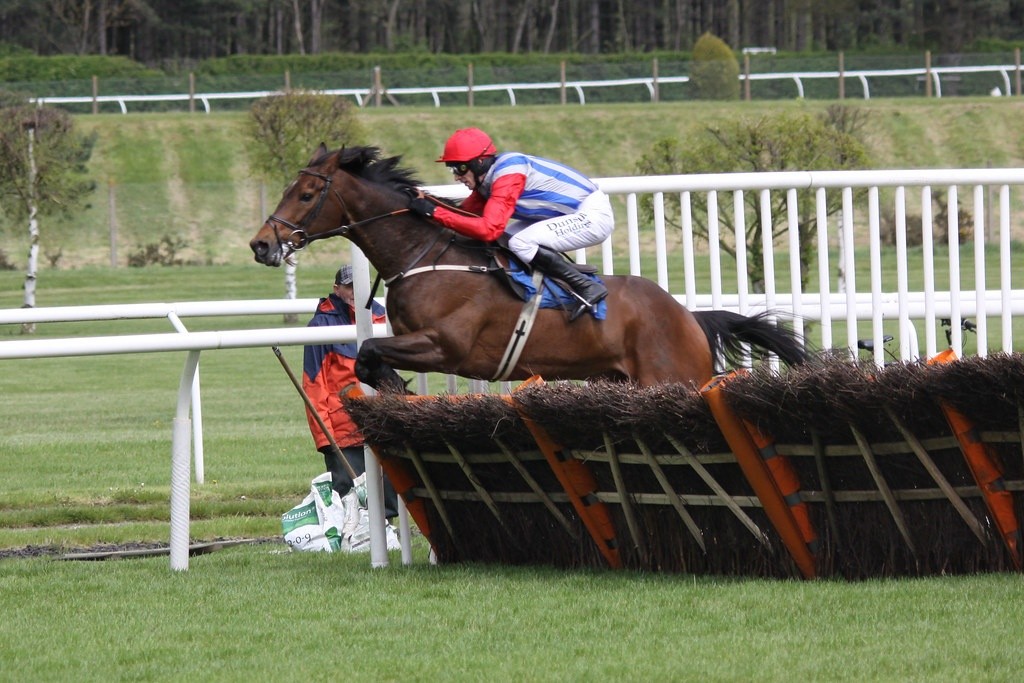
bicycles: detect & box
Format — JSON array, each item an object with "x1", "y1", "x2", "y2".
[{"x1": 831, "y1": 304, "x2": 981, "y2": 390}]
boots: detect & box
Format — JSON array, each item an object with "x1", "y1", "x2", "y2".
[{"x1": 528, "y1": 245, "x2": 608, "y2": 321}]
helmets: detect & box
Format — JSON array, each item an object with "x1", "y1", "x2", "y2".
[{"x1": 434, "y1": 128, "x2": 497, "y2": 162}]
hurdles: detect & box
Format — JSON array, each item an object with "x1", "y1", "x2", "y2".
[{"x1": 337, "y1": 348, "x2": 1024, "y2": 578}]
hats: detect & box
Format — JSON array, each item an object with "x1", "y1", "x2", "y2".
[{"x1": 336, "y1": 265, "x2": 353, "y2": 284}]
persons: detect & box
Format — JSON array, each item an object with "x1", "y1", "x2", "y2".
[
  {"x1": 406, "y1": 129, "x2": 614, "y2": 321},
  {"x1": 301, "y1": 265, "x2": 398, "y2": 526}
]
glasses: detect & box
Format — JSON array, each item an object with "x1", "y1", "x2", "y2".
[{"x1": 452, "y1": 164, "x2": 468, "y2": 176}]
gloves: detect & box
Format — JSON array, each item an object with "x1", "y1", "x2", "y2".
[
  {"x1": 318, "y1": 444, "x2": 343, "y2": 456},
  {"x1": 408, "y1": 198, "x2": 435, "y2": 219}
]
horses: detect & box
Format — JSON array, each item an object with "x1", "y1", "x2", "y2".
[{"x1": 249, "y1": 141, "x2": 818, "y2": 395}]
